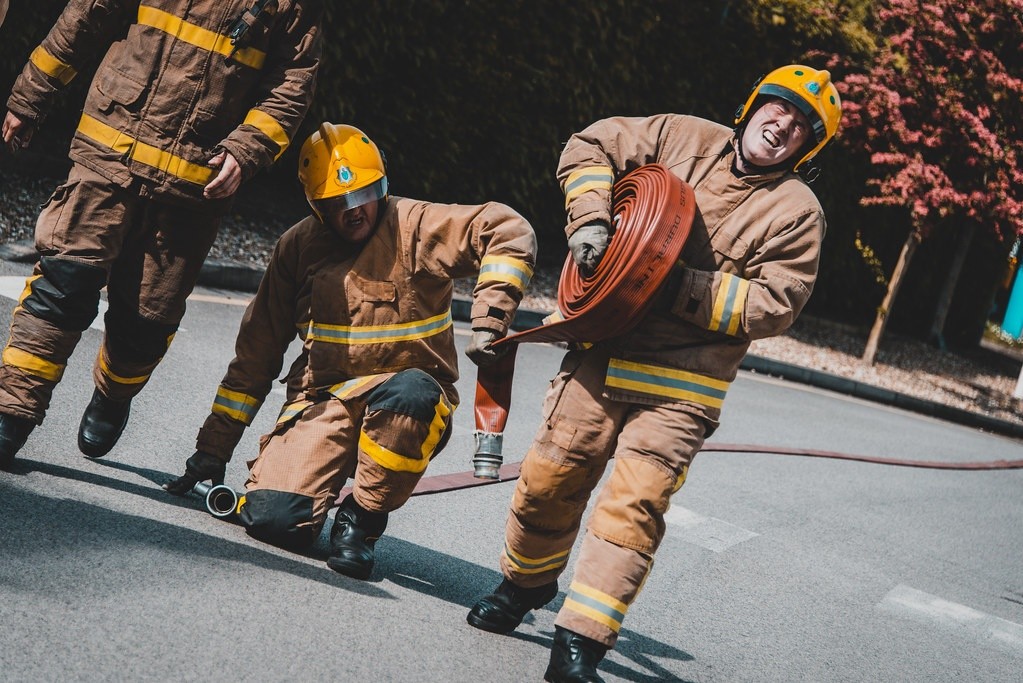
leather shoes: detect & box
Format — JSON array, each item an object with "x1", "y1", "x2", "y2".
[
  {"x1": 0, "y1": 411, "x2": 37, "y2": 471},
  {"x1": 467, "y1": 576, "x2": 558, "y2": 634},
  {"x1": 78, "y1": 386, "x2": 132, "y2": 458},
  {"x1": 544, "y1": 627, "x2": 608, "y2": 683},
  {"x1": 327, "y1": 492, "x2": 388, "y2": 579}
]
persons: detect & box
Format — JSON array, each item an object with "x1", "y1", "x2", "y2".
[
  {"x1": 465, "y1": 64, "x2": 842, "y2": 682},
  {"x1": 0, "y1": 0, "x2": 324, "y2": 469},
  {"x1": 167, "y1": 121, "x2": 538, "y2": 581}
]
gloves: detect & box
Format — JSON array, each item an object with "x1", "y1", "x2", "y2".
[
  {"x1": 465, "y1": 331, "x2": 509, "y2": 366},
  {"x1": 162, "y1": 450, "x2": 226, "y2": 497},
  {"x1": 568, "y1": 221, "x2": 610, "y2": 277}
]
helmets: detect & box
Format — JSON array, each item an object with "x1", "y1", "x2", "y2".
[
  {"x1": 734, "y1": 64, "x2": 842, "y2": 186},
  {"x1": 298, "y1": 121, "x2": 390, "y2": 225}
]
